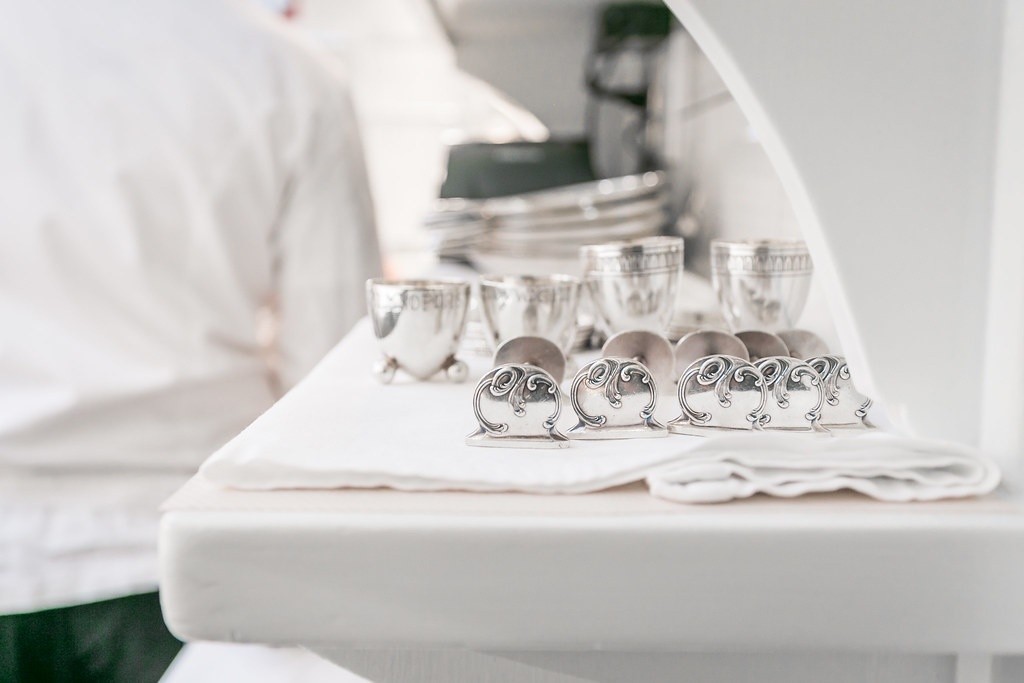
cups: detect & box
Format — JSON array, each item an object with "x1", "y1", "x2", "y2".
[
  {"x1": 579, "y1": 237, "x2": 684, "y2": 340},
  {"x1": 711, "y1": 238, "x2": 812, "y2": 332},
  {"x1": 366, "y1": 279, "x2": 471, "y2": 379},
  {"x1": 478, "y1": 272, "x2": 583, "y2": 362}
]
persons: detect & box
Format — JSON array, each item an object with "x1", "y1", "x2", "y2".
[{"x1": 0, "y1": 0, "x2": 385, "y2": 683}]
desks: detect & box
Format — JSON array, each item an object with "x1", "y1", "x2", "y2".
[{"x1": 156, "y1": 253, "x2": 1024, "y2": 658}]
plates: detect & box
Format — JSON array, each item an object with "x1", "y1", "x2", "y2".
[{"x1": 426, "y1": 172, "x2": 672, "y2": 269}]
